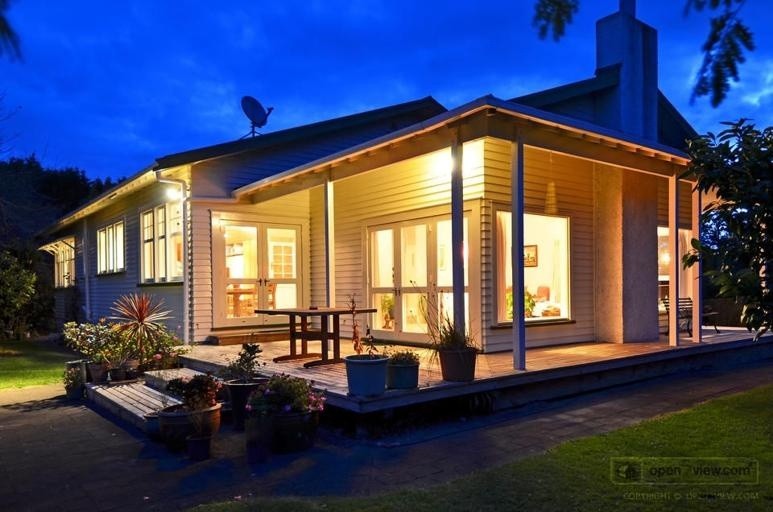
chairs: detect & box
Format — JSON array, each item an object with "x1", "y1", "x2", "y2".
[
  {"x1": 226, "y1": 277, "x2": 276, "y2": 318},
  {"x1": 660, "y1": 296, "x2": 721, "y2": 337},
  {"x1": 506, "y1": 285, "x2": 563, "y2": 318}
]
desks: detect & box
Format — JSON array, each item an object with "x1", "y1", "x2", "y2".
[{"x1": 254, "y1": 306, "x2": 378, "y2": 368}]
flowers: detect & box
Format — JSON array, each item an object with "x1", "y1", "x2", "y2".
[
  {"x1": 164, "y1": 371, "x2": 221, "y2": 411},
  {"x1": 152, "y1": 334, "x2": 190, "y2": 367},
  {"x1": 244, "y1": 371, "x2": 329, "y2": 420}
]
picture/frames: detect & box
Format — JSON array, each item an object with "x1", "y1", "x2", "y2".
[{"x1": 522, "y1": 244, "x2": 538, "y2": 267}]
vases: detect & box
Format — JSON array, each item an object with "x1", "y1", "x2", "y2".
[
  {"x1": 262, "y1": 410, "x2": 321, "y2": 455},
  {"x1": 164, "y1": 357, "x2": 175, "y2": 368},
  {"x1": 142, "y1": 402, "x2": 224, "y2": 461}
]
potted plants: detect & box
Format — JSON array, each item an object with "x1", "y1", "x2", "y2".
[
  {"x1": 216, "y1": 330, "x2": 270, "y2": 432},
  {"x1": 340, "y1": 280, "x2": 478, "y2": 398},
  {"x1": 64, "y1": 290, "x2": 163, "y2": 402}
]
